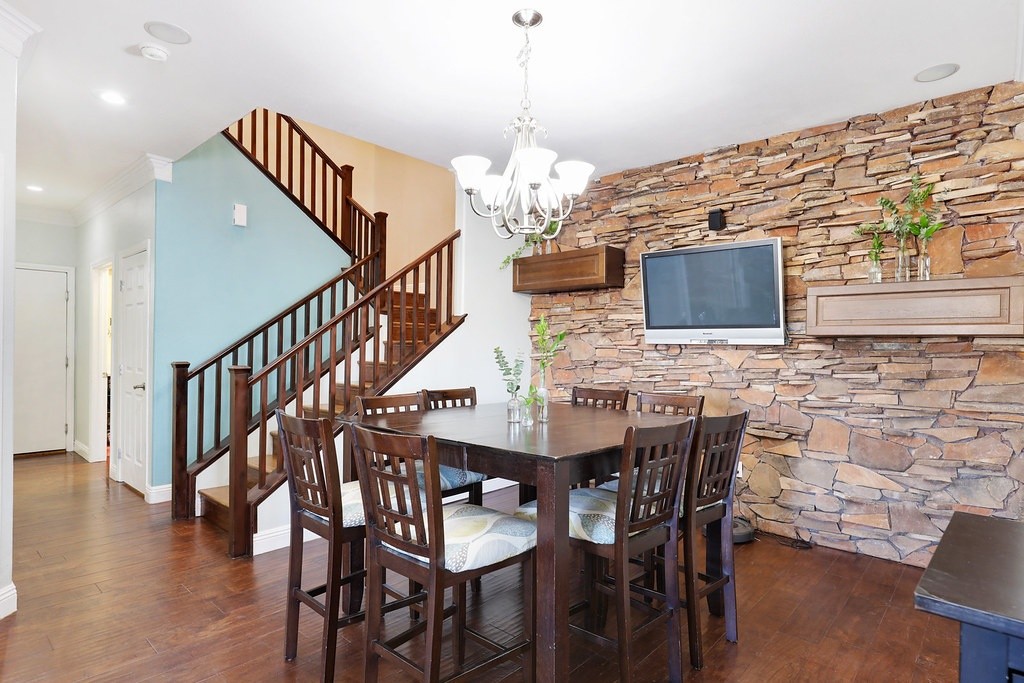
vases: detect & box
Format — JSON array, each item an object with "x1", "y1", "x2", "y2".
[
  {"x1": 532, "y1": 241, "x2": 542, "y2": 256},
  {"x1": 918, "y1": 249, "x2": 930, "y2": 281},
  {"x1": 895, "y1": 239, "x2": 911, "y2": 283},
  {"x1": 868, "y1": 259, "x2": 883, "y2": 284},
  {"x1": 520, "y1": 404, "x2": 534, "y2": 426},
  {"x1": 546, "y1": 240, "x2": 551, "y2": 255},
  {"x1": 537, "y1": 369, "x2": 548, "y2": 424},
  {"x1": 507, "y1": 393, "x2": 520, "y2": 423}
]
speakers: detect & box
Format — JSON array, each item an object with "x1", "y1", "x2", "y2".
[{"x1": 708, "y1": 212, "x2": 726, "y2": 231}]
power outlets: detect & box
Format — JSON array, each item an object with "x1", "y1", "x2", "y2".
[{"x1": 735, "y1": 461, "x2": 742, "y2": 478}]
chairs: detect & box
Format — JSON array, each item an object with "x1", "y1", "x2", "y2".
[{"x1": 273, "y1": 386, "x2": 752, "y2": 683}]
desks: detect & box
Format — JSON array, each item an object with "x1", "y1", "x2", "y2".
[
  {"x1": 913, "y1": 511, "x2": 1024, "y2": 683},
  {"x1": 335, "y1": 401, "x2": 696, "y2": 683}
]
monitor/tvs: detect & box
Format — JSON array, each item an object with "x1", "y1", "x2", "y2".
[{"x1": 639, "y1": 237, "x2": 786, "y2": 346}]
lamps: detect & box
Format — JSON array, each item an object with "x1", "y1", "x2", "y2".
[{"x1": 449, "y1": 8, "x2": 596, "y2": 241}]
